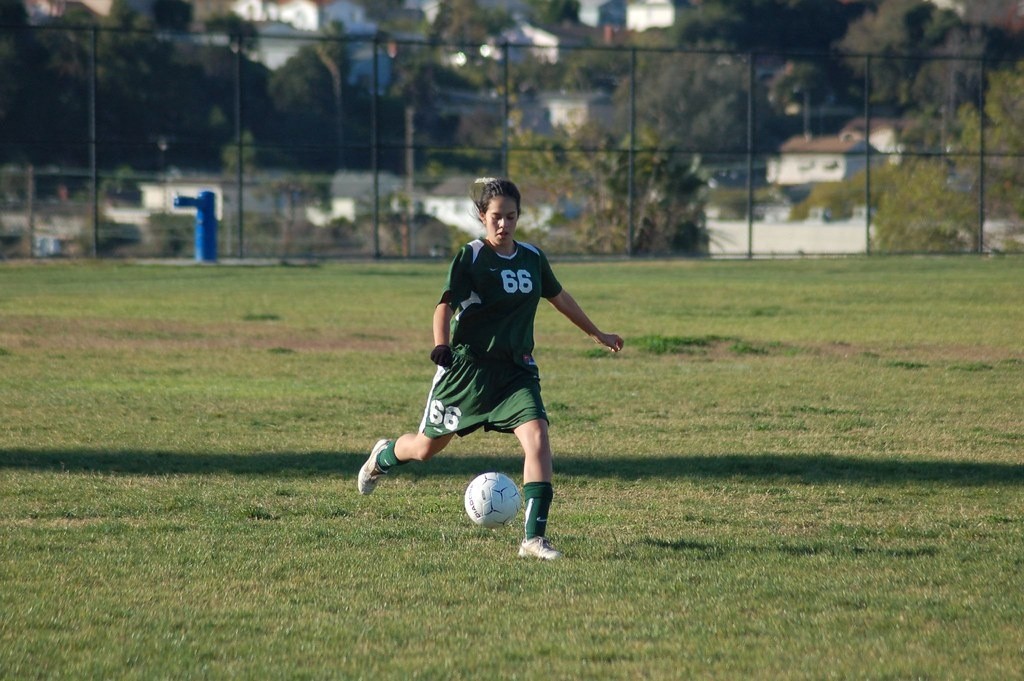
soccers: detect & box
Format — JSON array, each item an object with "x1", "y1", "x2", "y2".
[{"x1": 463, "y1": 471, "x2": 521, "y2": 529}]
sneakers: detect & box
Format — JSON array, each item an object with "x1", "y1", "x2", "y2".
[
  {"x1": 358, "y1": 439, "x2": 390, "y2": 494},
  {"x1": 518, "y1": 539, "x2": 563, "y2": 562}
]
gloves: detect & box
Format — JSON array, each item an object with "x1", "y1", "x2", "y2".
[{"x1": 431, "y1": 345, "x2": 454, "y2": 370}]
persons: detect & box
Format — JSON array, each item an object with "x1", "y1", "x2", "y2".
[{"x1": 356, "y1": 175, "x2": 625, "y2": 562}]
